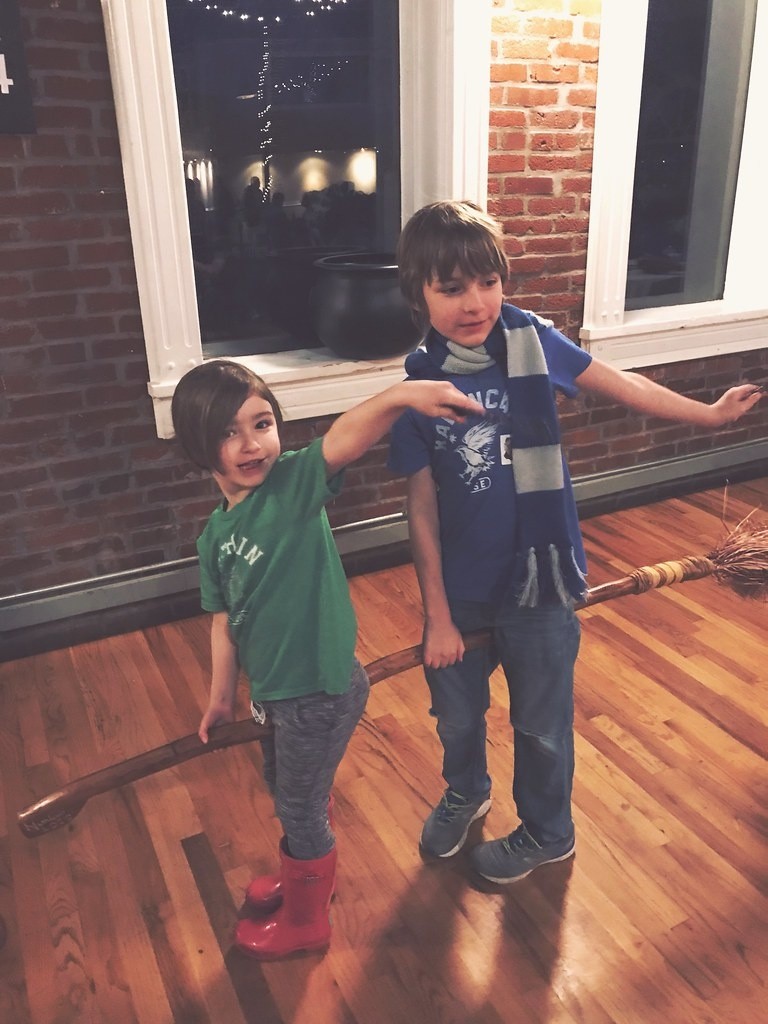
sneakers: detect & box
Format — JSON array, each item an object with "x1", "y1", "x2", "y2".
[
  {"x1": 420, "y1": 786, "x2": 493, "y2": 858},
  {"x1": 468, "y1": 823, "x2": 576, "y2": 884}
]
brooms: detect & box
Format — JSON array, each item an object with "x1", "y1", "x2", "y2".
[{"x1": 17, "y1": 478, "x2": 768, "y2": 839}]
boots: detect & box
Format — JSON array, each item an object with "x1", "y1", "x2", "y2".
[
  {"x1": 244, "y1": 792, "x2": 339, "y2": 909},
  {"x1": 236, "y1": 835, "x2": 337, "y2": 960}
]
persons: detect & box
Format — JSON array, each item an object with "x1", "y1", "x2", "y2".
[
  {"x1": 170, "y1": 358, "x2": 488, "y2": 962},
  {"x1": 186, "y1": 175, "x2": 379, "y2": 289},
  {"x1": 385, "y1": 198, "x2": 768, "y2": 886}
]
